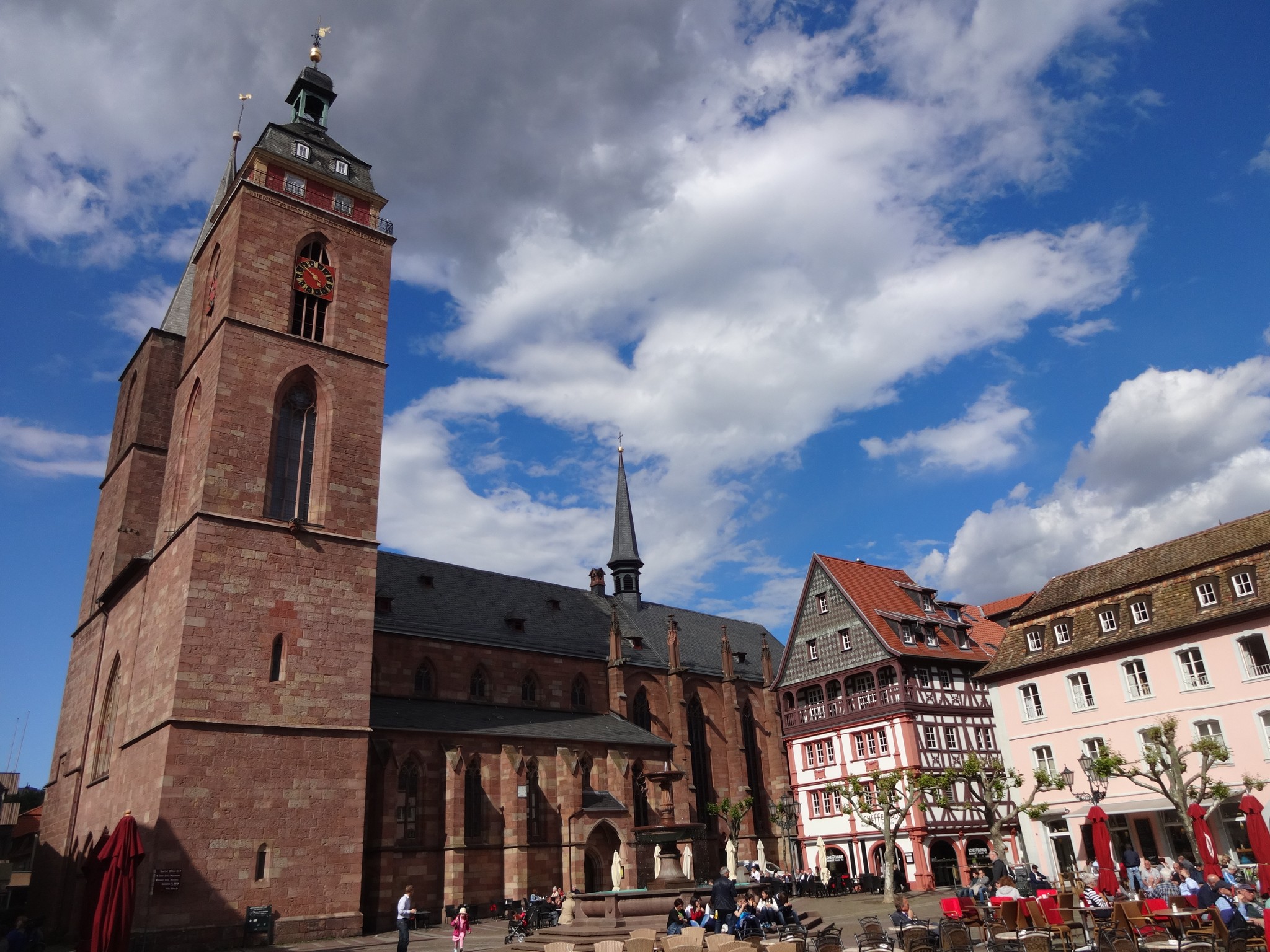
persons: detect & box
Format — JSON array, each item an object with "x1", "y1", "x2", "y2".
[
  {"x1": 451, "y1": 908, "x2": 472, "y2": 952},
  {"x1": 894, "y1": 843, "x2": 1270, "y2": 952},
  {"x1": 6, "y1": 915, "x2": 41, "y2": 952},
  {"x1": 397, "y1": 886, "x2": 417, "y2": 952},
  {"x1": 530, "y1": 863, "x2": 820, "y2": 938}
]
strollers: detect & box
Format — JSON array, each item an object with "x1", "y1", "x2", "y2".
[{"x1": 504, "y1": 900, "x2": 542, "y2": 945}]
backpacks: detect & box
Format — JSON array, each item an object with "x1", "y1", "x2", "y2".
[
  {"x1": 1234, "y1": 869, "x2": 1245, "y2": 882},
  {"x1": 993, "y1": 860, "x2": 1018, "y2": 884}
]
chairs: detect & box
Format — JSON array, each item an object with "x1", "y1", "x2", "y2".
[{"x1": 542, "y1": 872, "x2": 1270, "y2": 952}]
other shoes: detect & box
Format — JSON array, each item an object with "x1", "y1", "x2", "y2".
[
  {"x1": 785, "y1": 927, "x2": 792, "y2": 932},
  {"x1": 797, "y1": 927, "x2": 808, "y2": 934},
  {"x1": 721, "y1": 924, "x2": 728, "y2": 932},
  {"x1": 763, "y1": 923, "x2": 771, "y2": 928},
  {"x1": 1241, "y1": 879, "x2": 1247, "y2": 884},
  {"x1": 454, "y1": 948, "x2": 457, "y2": 952},
  {"x1": 459, "y1": 947, "x2": 463, "y2": 952}
]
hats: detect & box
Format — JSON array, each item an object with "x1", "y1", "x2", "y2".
[
  {"x1": 1239, "y1": 884, "x2": 1257, "y2": 892},
  {"x1": 459, "y1": 908, "x2": 468, "y2": 914},
  {"x1": 1214, "y1": 880, "x2": 1232, "y2": 890},
  {"x1": 1196, "y1": 861, "x2": 1202, "y2": 867}
]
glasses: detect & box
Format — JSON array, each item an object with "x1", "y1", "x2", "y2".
[
  {"x1": 1246, "y1": 891, "x2": 1255, "y2": 894},
  {"x1": 1173, "y1": 867, "x2": 1178, "y2": 869},
  {"x1": 678, "y1": 905, "x2": 684, "y2": 908},
  {"x1": 728, "y1": 871, "x2": 730, "y2": 873},
  {"x1": 696, "y1": 900, "x2": 703, "y2": 904}
]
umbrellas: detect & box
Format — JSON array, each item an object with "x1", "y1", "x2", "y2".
[
  {"x1": 611, "y1": 835, "x2": 831, "y2": 899},
  {"x1": 80, "y1": 809, "x2": 143, "y2": 952},
  {"x1": 1087, "y1": 795, "x2": 1270, "y2": 909}
]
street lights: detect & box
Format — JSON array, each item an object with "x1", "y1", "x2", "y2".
[
  {"x1": 767, "y1": 791, "x2": 802, "y2": 898},
  {"x1": 1059, "y1": 749, "x2": 1111, "y2": 807}
]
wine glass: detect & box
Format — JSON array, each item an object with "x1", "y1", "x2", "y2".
[{"x1": 1127, "y1": 889, "x2": 1133, "y2": 901}]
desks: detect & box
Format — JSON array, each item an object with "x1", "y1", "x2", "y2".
[
  {"x1": 1047, "y1": 905, "x2": 1114, "y2": 952},
  {"x1": 994, "y1": 930, "x2": 1054, "y2": 941},
  {"x1": 964, "y1": 904, "x2": 1012, "y2": 950},
  {"x1": 1151, "y1": 907, "x2": 1205, "y2": 938},
  {"x1": 759, "y1": 937, "x2": 812, "y2": 952},
  {"x1": 1144, "y1": 940, "x2": 1193, "y2": 952},
  {"x1": 887, "y1": 924, "x2": 938, "y2": 931}
]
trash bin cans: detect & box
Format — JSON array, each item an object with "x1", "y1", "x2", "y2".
[{"x1": 244, "y1": 904, "x2": 272, "y2": 932}]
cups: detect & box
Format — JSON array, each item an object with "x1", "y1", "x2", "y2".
[
  {"x1": 1233, "y1": 896, "x2": 1238, "y2": 902},
  {"x1": 912, "y1": 913, "x2": 918, "y2": 920},
  {"x1": 1020, "y1": 930, "x2": 1026, "y2": 937},
  {"x1": 1133, "y1": 893, "x2": 1139, "y2": 900},
  {"x1": 1171, "y1": 903, "x2": 1178, "y2": 912},
  {"x1": 987, "y1": 900, "x2": 992, "y2": 906}
]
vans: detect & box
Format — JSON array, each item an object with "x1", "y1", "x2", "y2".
[{"x1": 737, "y1": 860, "x2": 792, "y2": 882}]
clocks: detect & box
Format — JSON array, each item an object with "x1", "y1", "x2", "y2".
[{"x1": 292, "y1": 254, "x2": 336, "y2": 302}]
